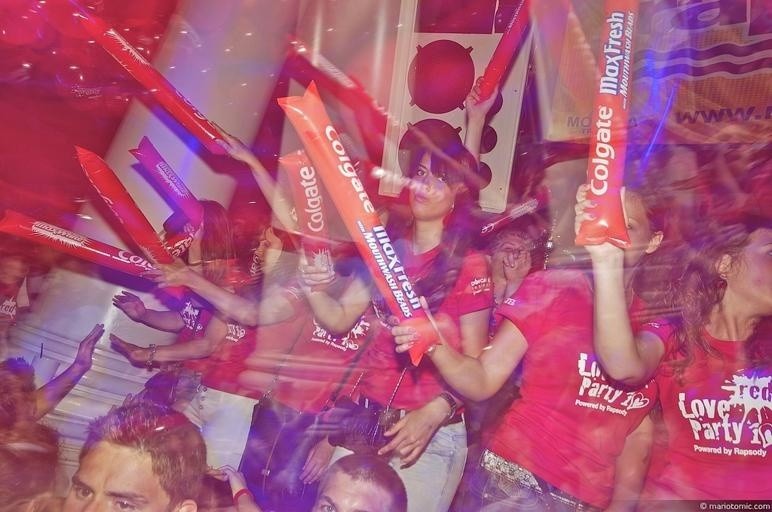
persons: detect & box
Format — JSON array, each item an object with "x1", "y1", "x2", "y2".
[{"x1": 0, "y1": 116, "x2": 772, "y2": 511}]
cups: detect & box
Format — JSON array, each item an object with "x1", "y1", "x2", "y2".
[{"x1": 30, "y1": 354, "x2": 62, "y2": 391}]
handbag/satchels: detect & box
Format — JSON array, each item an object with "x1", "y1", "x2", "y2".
[
  {"x1": 324, "y1": 396, "x2": 407, "y2": 464},
  {"x1": 232, "y1": 393, "x2": 327, "y2": 502},
  {"x1": 143, "y1": 369, "x2": 180, "y2": 410}
]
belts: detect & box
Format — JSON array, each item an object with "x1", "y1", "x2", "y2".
[
  {"x1": 476, "y1": 447, "x2": 595, "y2": 511},
  {"x1": 442, "y1": 410, "x2": 467, "y2": 428}
]
